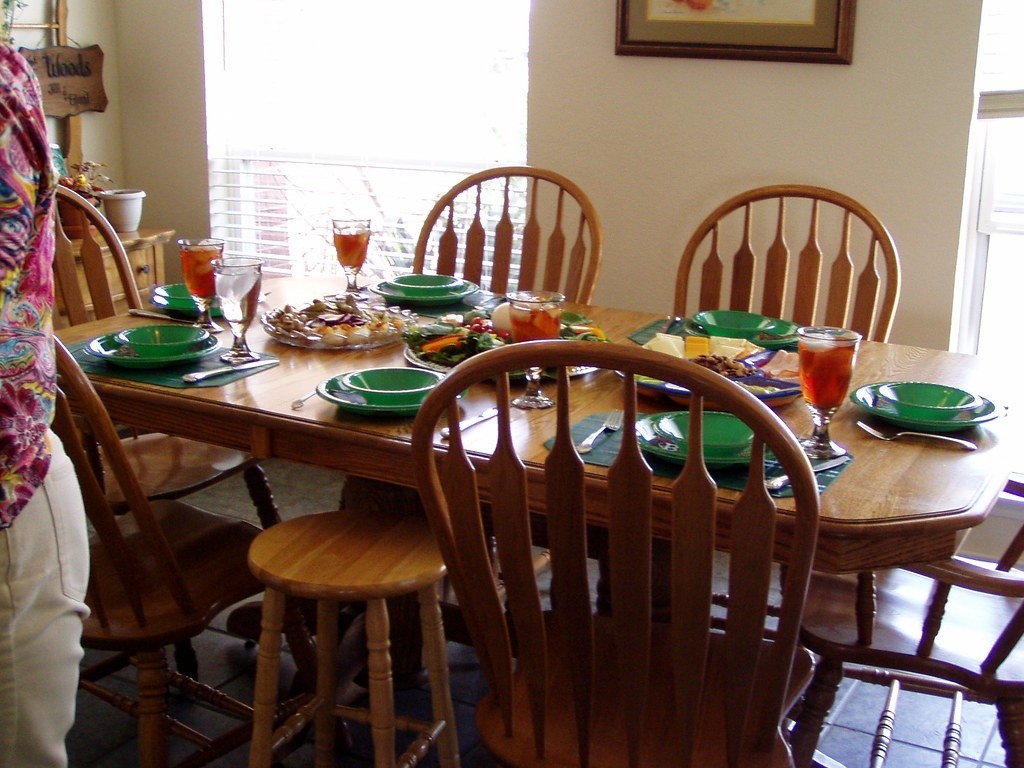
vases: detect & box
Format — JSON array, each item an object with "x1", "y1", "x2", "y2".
[
  {"x1": 59, "y1": 198, "x2": 99, "y2": 237},
  {"x1": 98, "y1": 189, "x2": 146, "y2": 231}
]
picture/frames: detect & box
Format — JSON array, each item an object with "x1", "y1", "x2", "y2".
[{"x1": 616, "y1": 0, "x2": 858, "y2": 65}]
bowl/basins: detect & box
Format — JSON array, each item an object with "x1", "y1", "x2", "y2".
[
  {"x1": 385, "y1": 274, "x2": 464, "y2": 296},
  {"x1": 691, "y1": 308, "x2": 777, "y2": 339},
  {"x1": 341, "y1": 366, "x2": 441, "y2": 406},
  {"x1": 876, "y1": 381, "x2": 984, "y2": 420},
  {"x1": 114, "y1": 322, "x2": 209, "y2": 357},
  {"x1": 652, "y1": 410, "x2": 755, "y2": 456},
  {"x1": 152, "y1": 282, "x2": 218, "y2": 308}
]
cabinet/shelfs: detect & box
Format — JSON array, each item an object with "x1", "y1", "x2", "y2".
[{"x1": 50, "y1": 227, "x2": 177, "y2": 331}]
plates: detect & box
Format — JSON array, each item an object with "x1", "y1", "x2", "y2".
[
  {"x1": 369, "y1": 281, "x2": 480, "y2": 308},
  {"x1": 260, "y1": 301, "x2": 419, "y2": 349},
  {"x1": 83, "y1": 331, "x2": 220, "y2": 369},
  {"x1": 849, "y1": 381, "x2": 1006, "y2": 431},
  {"x1": 149, "y1": 295, "x2": 223, "y2": 319},
  {"x1": 614, "y1": 348, "x2": 802, "y2": 410},
  {"x1": 313, "y1": 370, "x2": 467, "y2": 413},
  {"x1": 634, "y1": 411, "x2": 776, "y2": 463},
  {"x1": 402, "y1": 343, "x2": 600, "y2": 380},
  {"x1": 682, "y1": 318, "x2": 801, "y2": 349}
]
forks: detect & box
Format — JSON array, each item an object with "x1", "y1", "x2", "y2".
[
  {"x1": 576, "y1": 409, "x2": 623, "y2": 453},
  {"x1": 855, "y1": 420, "x2": 977, "y2": 450}
]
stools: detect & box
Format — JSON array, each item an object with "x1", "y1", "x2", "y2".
[{"x1": 245, "y1": 511, "x2": 461, "y2": 768}]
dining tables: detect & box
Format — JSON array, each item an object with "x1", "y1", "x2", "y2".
[{"x1": 50, "y1": 271, "x2": 1024, "y2": 768}]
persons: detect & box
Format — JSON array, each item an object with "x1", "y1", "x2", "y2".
[{"x1": 0, "y1": 40, "x2": 91, "y2": 768}]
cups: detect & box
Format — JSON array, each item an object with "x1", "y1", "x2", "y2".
[{"x1": 99, "y1": 188, "x2": 147, "y2": 232}]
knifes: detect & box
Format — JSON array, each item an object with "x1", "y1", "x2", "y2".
[
  {"x1": 182, "y1": 359, "x2": 280, "y2": 382},
  {"x1": 653, "y1": 315, "x2": 676, "y2": 339}
]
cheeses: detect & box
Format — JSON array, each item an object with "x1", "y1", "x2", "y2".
[{"x1": 644, "y1": 332, "x2": 746, "y2": 361}]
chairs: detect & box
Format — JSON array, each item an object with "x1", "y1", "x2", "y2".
[{"x1": 45, "y1": 166, "x2": 1024, "y2": 768}]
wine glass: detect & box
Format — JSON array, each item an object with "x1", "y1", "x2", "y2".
[
  {"x1": 211, "y1": 257, "x2": 264, "y2": 364},
  {"x1": 332, "y1": 218, "x2": 371, "y2": 301},
  {"x1": 177, "y1": 237, "x2": 225, "y2": 333},
  {"x1": 504, "y1": 289, "x2": 565, "y2": 408},
  {"x1": 796, "y1": 326, "x2": 862, "y2": 459}
]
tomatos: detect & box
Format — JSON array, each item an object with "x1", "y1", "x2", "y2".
[{"x1": 470, "y1": 317, "x2": 509, "y2": 338}]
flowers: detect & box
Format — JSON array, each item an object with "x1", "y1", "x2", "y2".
[{"x1": 59, "y1": 161, "x2": 113, "y2": 200}]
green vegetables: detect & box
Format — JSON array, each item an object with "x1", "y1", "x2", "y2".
[
  {"x1": 559, "y1": 310, "x2": 612, "y2": 343},
  {"x1": 402, "y1": 322, "x2": 492, "y2": 366}
]
